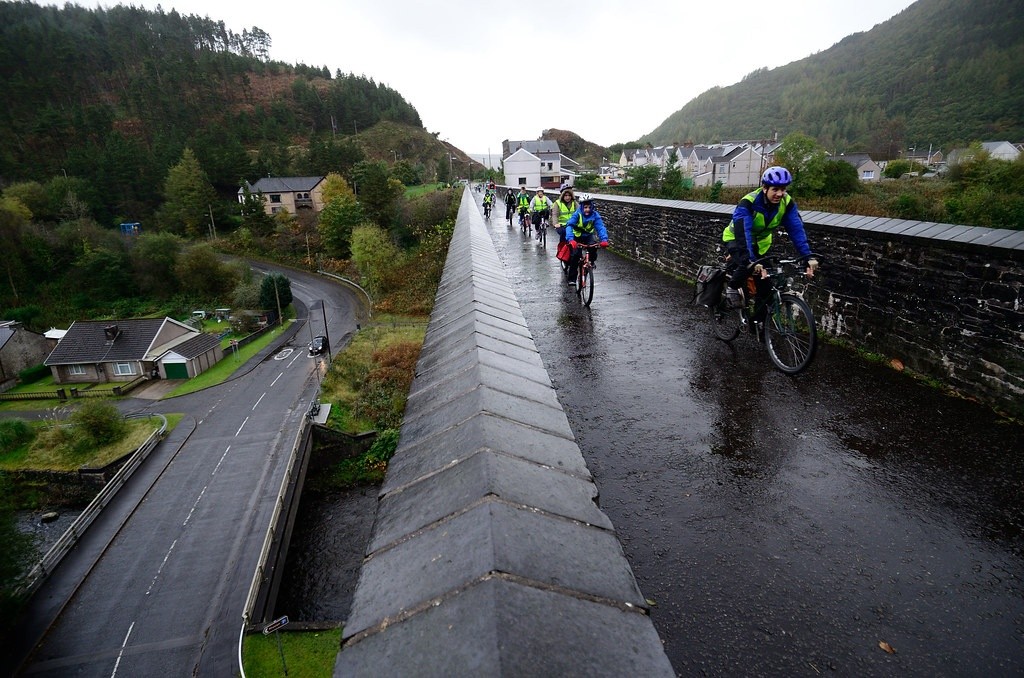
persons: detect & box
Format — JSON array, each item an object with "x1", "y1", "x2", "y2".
[
  {"x1": 723, "y1": 166, "x2": 818, "y2": 338},
  {"x1": 504, "y1": 188, "x2": 516, "y2": 222},
  {"x1": 566, "y1": 193, "x2": 609, "y2": 285},
  {"x1": 487, "y1": 179, "x2": 497, "y2": 204},
  {"x1": 516, "y1": 186, "x2": 532, "y2": 231},
  {"x1": 483, "y1": 192, "x2": 492, "y2": 215},
  {"x1": 530, "y1": 186, "x2": 553, "y2": 240},
  {"x1": 477, "y1": 180, "x2": 482, "y2": 190},
  {"x1": 552, "y1": 184, "x2": 580, "y2": 246}
]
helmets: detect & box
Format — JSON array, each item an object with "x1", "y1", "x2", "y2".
[
  {"x1": 761, "y1": 167, "x2": 793, "y2": 186},
  {"x1": 536, "y1": 187, "x2": 544, "y2": 191},
  {"x1": 560, "y1": 183, "x2": 573, "y2": 193},
  {"x1": 578, "y1": 193, "x2": 595, "y2": 203}
]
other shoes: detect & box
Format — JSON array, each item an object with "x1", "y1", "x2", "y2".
[
  {"x1": 535, "y1": 231, "x2": 539, "y2": 240},
  {"x1": 545, "y1": 219, "x2": 549, "y2": 228},
  {"x1": 757, "y1": 321, "x2": 774, "y2": 343},
  {"x1": 694, "y1": 265, "x2": 727, "y2": 308},
  {"x1": 589, "y1": 263, "x2": 596, "y2": 270},
  {"x1": 722, "y1": 285, "x2": 744, "y2": 309},
  {"x1": 569, "y1": 280, "x2": 576, "y2": 286}
]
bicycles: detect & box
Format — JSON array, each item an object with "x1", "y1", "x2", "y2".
[
  {"x1": 484, "y1": 195, "x2": 497, "y2": 219},
  {"x1": 504, "y1": 204, "x2": 553, "y2": 249},
  {"x1": 708, "y1": 244, "x2": 824, "y2": 375},
  {"x1": 553, "y1": 224, "x2": 614, "y2": 306}
]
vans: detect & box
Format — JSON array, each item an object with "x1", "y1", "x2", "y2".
[{"x1": 459, "y1": 180, "x2": 468, "y2": 185}]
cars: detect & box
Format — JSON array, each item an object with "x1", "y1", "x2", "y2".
[{"x1": 307, "y1": 336, "x2": 328, "y2": 355}]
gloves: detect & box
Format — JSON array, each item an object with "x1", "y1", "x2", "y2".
[
  {"x1": 600, "y1": 242, "x2": 608, "y2": 248},
  {"x1": 569, "y1": 240, "x2": 577, "y2": 249}
]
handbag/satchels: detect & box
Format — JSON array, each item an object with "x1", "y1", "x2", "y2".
[{"x1": 557, "y1": 242, "x2": 570, "y2": 261}]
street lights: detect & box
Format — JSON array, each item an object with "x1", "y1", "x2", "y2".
[
  {"x1": 310, "y1": 298, "x2": 332, "y2": 363},
  {"x1": 447, "y1": 152, "x2": 457, "y2": 172},
  {"x1": 483, "y1": 158, "x2": 487, "y2": 179},
  {"x1": 469, "y1": 163, "x2": 474, "y2": 191},
  {"x1": 288, "y1": 318, "x2": 319, "y2": 383}
]
